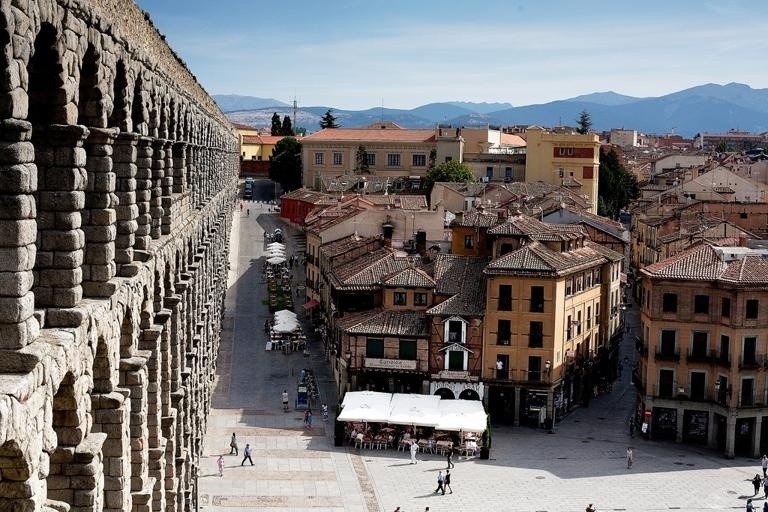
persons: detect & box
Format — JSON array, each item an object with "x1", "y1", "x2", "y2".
[
  {"x1": 217, "y1": 456, "x2": 224, "y2": 476},
  {"x1": 626, "y1": 448, "x2": 632, "y2": 469},
  {"x1": 424, "y1": 507, "x2": 429, "y2": 512},
  {"x1": 343, "y1": 418, "x2": 455, "y2": 469},
  {"x1": 434, "y1": 471, "x2": 444, "y2": 495},
  {"x1": 592, "y1": 355, "x2": 629, "y2": 400},
  {"x1": 240, "y1": 202, "x2": 243, "y2": 211},
  {"x1": 586, "y1": 504, "x2": 596, "y2": 512},
  {"x1": 230, "y1": 433, "x2": 238, "y2": 456},
  {"x1": 746, "y1": 454, "x2": 768, "y2": 512},
  {"x1": 394, "y1": 507, "x2": 401, "y2": 512},
  {"x1": 263, "y1": 228, "x2": 311, "y2": 357},
  {"x1": 282, "y1": 368, "x2": 329, "y2": 429},
  {"x1": 495, "y1": 359, "x2": 504, "y2": 380},
  {"x1": 247, "y1": 208, "x2": 250, "y2": 216},
  {"x1": 242, "y1": 444, "x2": 255, "y2": 466},
  {"x1": 444, "y1": 470, "x2": 453, "y2": 494}
]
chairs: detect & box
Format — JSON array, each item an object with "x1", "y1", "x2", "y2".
[
  {"x1": 349, "y1": 430, "x2": 453, "y2": 456},
  {"x1": 457, "y1": 431, "x2": 481, "y2": 459}
]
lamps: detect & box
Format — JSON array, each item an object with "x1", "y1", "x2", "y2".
[
  {"x1": 545, "y1": 359, "x2": 551, "y2": 368},
  {"x1": 345, "y1": 350, "x2": 352, "y2": 359},
  {"x1": 714, "y1": 379, "x2": 732, "y2": 401}
]
